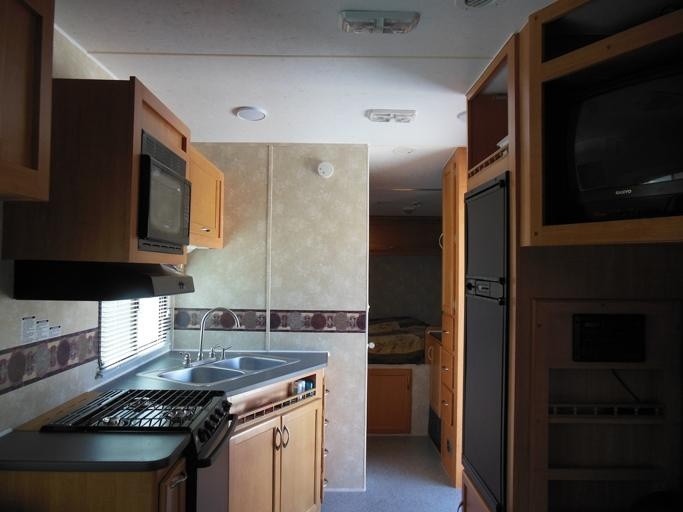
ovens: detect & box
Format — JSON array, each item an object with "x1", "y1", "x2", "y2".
[{"x1": 189, "y1": 394, "x2": 240, "y2": 512}]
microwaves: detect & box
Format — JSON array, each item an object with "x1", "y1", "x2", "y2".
[{"x1": 135, "y1": 129, "x2": 195, "y2": 255}]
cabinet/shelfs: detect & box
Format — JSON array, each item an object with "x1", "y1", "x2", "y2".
[
  {"x1": 439, "y1": 147, "x2": 470, "y2": 490},
  {"x1": 425, "y1": 331, "x2": 441, "y2": 451},
  {"x1": 367, "y1": 368, "x2": 413, "y2": 435},
  {"x1": 157, "y1": 456, "x2": 187, "y2": 512},
  {"x1": 227, "y1": 399, "x2": 324, "y2": 512},
  {"x1": 0, "y1": 0, "x2": 55, "y2": 205},
  {"x1": 188, "y1": 143, "x2": 223, "y2": 248},
  {"x1": 0, "y1": 74, "x2": 191, "y2": 266},
  {"x1": 464, "y1": 0, "x2": 683, "y2": 248}
]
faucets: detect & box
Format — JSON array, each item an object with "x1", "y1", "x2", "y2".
[{"x1": 199, "y1": 307, "x2": 241, "y2": 353}]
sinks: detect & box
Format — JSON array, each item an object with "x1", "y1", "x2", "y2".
[
  {"x1": 211, "y1": 357, "x2": 301, "y2": 372},
  {"x1": 135, "y1": 366, "x2": 244, "y2": 387}
]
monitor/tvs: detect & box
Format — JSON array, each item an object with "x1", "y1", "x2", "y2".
[{"x1": 554, "y1": 68, "x2": 680, "y2": 215}]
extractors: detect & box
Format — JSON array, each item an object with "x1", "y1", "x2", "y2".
[{"x1": 11, "y1": 264, "x2": 196, "y2": 302}]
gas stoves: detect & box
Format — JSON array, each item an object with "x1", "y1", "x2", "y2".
[{"x1": 36, "y1": 386, "x2": 217, "y2": 431}]
